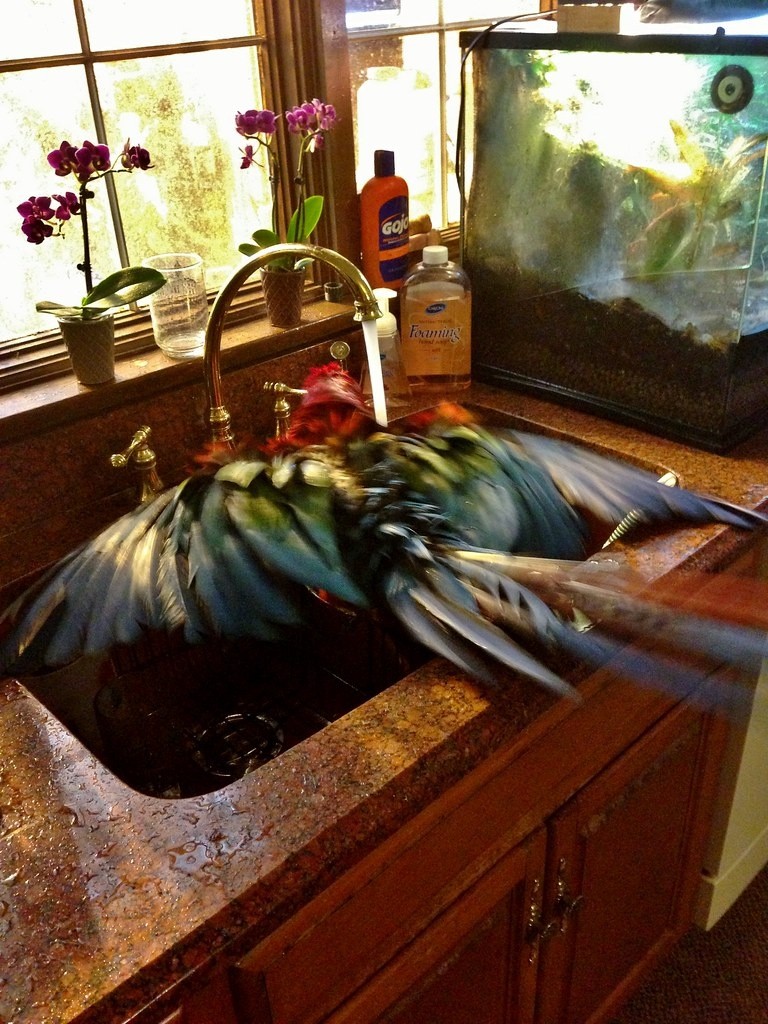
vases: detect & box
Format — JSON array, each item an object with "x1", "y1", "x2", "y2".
[
  {"x1": 58, "y1": 311, "x2": 114, "y2": 386},
  {"x1": 259, "y1": 265, "x2": 306, "y2": 327}
]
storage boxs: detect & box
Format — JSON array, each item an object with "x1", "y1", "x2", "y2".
[{"x1": 460, "y1": 0, "x2": 768, "y2": 456}]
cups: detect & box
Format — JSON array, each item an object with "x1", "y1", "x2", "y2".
[{"x1": 142, "y1": 253, "x2": 209, "y2": 359}]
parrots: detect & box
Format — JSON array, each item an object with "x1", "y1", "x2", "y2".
[{"x1": 0, "y1": 363, "x2": 768, "y2": 722}]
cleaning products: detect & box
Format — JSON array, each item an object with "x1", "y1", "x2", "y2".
[{"x1": 359, "y1": 150, "x2": 408, "y2": 292}]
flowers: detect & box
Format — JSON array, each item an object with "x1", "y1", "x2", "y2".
[
  {"x1": 16, "y1": 137, "x2": 166, "y2": 319},
  {"x1": 236, "y1": 98, "x2": 337, "y2": 270}
]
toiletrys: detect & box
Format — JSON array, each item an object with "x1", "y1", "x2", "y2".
[{"x1": 399, "y1": 245, "x2": 472, "y2": 395}]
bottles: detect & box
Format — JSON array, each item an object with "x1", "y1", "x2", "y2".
[
  {"x1": 361, "y1": 150, "x2": 409, "y2": 289},
  {"x1": 401, "y1": 246, "x2": 471, "y2": 397}
]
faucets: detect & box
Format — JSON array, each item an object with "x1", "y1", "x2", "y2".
[{"x1": 203, "y1": 242, "x2": 384, "y2": 458}]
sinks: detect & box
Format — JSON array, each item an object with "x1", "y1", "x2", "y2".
[{"x1": 0, "y1": 396, "x2": 687, "y2": 802}]
